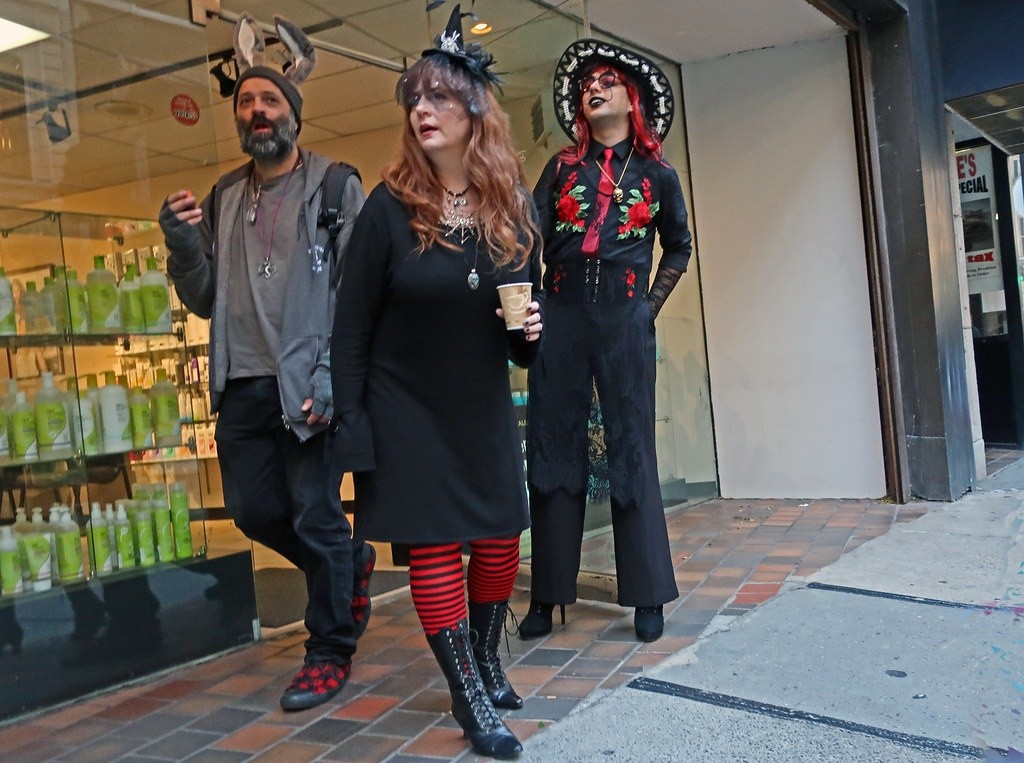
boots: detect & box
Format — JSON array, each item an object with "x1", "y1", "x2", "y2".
[
  {"x1": 425, "y1": 617, "x2": 523, "y2": 758},
  {"x1": 466, "y1": 598, "x2": 524, "y2": 710}
]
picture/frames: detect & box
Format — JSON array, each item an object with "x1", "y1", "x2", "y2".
[{"x1": 3, "y1": 262, "x2": 65, "y2": 380}]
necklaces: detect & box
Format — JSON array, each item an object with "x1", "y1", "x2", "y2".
[
  {"x1": 596, "y1": 147, "x2": 634, "y2": 203},
  {"x1": 253, "y1": 153, "x2": 301, "y2": 279},
  {"x1": 247, "y1": 174, "x2": 261, "y2": 223},
  {"x1": 442, "y1": 184, "x2": 479, "y2": 291}
]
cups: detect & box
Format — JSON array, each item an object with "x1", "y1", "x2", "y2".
[{"x1": 496, "y1": 282, "x2": 534, "y2": 330}]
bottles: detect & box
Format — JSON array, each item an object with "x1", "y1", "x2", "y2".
[
  {"x1": 1, "y1": 371, "x2": 183, "y2": 466},
  {"x1": 0, "y1": 254, "x2": 172, "y2": 338},
  {"x1": 0, "y1": 483, "x2": 193, "y2": 600}
]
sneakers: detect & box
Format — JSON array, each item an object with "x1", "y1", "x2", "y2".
[
  {"x1": 281, "y1": 656, "x2": 351, "y2": 708},
  {"x1": 351, "y1": 544, "x2": 376, "y2": 639}
]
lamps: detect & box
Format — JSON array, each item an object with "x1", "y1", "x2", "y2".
[
  {"x1": 210, "y1": 54, "x2": 242, "y2": 101},
  {"x1": 33, "y1": 104, "x2": 72, "y2": 144},
  {"x1": 459, "y1": 12, "x2": 493, "y2": 35}
]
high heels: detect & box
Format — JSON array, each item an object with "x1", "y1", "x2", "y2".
[
  {"x1": 634, "y1": 604, "x2": 664, "y2": 643},
  {"x1": 518, "y1": 598, "x2": 566, "y2": 640}
]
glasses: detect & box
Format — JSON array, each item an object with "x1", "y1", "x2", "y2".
[{"x1": 580, "y1": 71, "x2": 627, "y2": 93}]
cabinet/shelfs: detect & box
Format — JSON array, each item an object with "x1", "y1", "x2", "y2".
[
  {"x1": 0, "y1": 204, "x2": 211, "y2": 602},
  {"x1": 107, "y1": 244, "x2": 355, "y2": 510}
]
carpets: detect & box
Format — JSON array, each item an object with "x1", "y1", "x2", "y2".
[{"x1": 252, "y1": 567, "x2": 410, "y2": 629}]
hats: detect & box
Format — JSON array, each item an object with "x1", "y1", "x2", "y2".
[
  {"x1": 231, "y1": 13, "x2": 317, "y2": 135},
  {"x1": 552, "y1": 38, "x2": 674, "y2": 151}
]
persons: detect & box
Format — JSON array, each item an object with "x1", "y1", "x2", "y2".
[
  {"x1": 518, "y1": 38, "x2": 693, "y2": 643},
  {"x1": 158, "y1": 68, "x2": 374, "y2": 709},
  {"x1": 329, "y1": 5, "x2": 546, "y2": 756}
]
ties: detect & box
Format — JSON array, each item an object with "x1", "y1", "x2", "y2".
[{"x1": 582, "y1": 147, "x2": 615, "y2": 254}]
